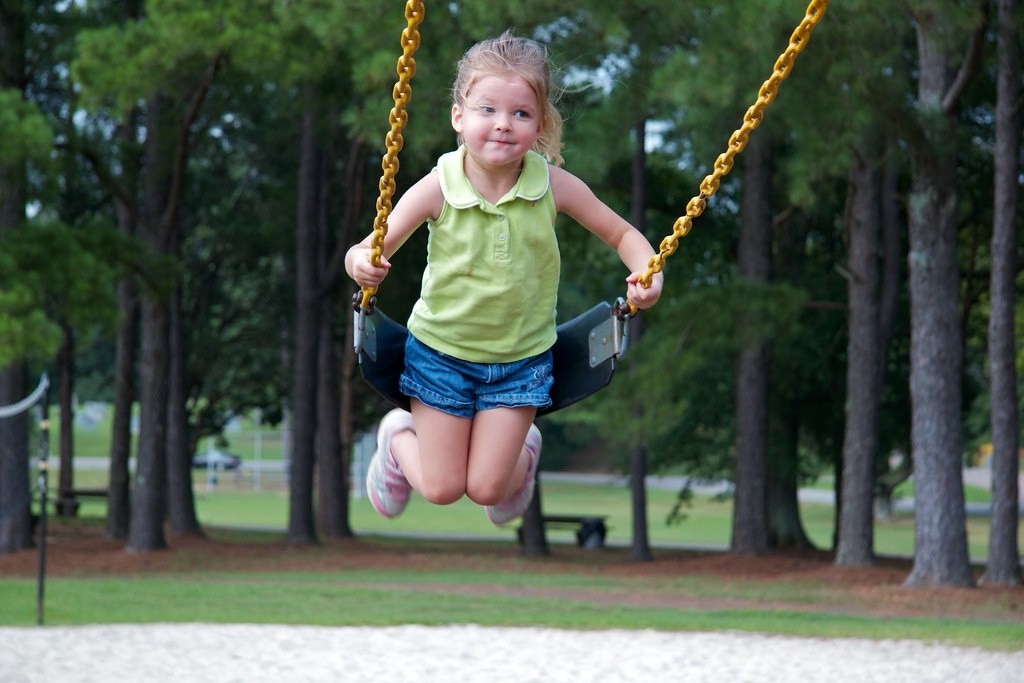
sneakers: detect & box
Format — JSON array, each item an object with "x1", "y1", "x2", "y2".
[
  {"x1": 486, "y1": 423, "x2": 542, "y2": 526},
  {"x1": 366, "y1": 407, "x2": 417, "y2": 519}
]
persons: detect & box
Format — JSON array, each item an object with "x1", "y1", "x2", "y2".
[{"x1": 345, "y1": 29, "x2": 664, "y2": 526}]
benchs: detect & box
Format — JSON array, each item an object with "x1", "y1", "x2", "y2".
[
  {"x1": 513, "y1": 514, "x2": 610, "y2": 551},
  {"x1": 57, "y1": 486, "x2": 112, "y2": 517}
]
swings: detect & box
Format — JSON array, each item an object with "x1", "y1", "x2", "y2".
[{"x1": 351, "y1": 2, "x2": 824, "y2": 415}]
